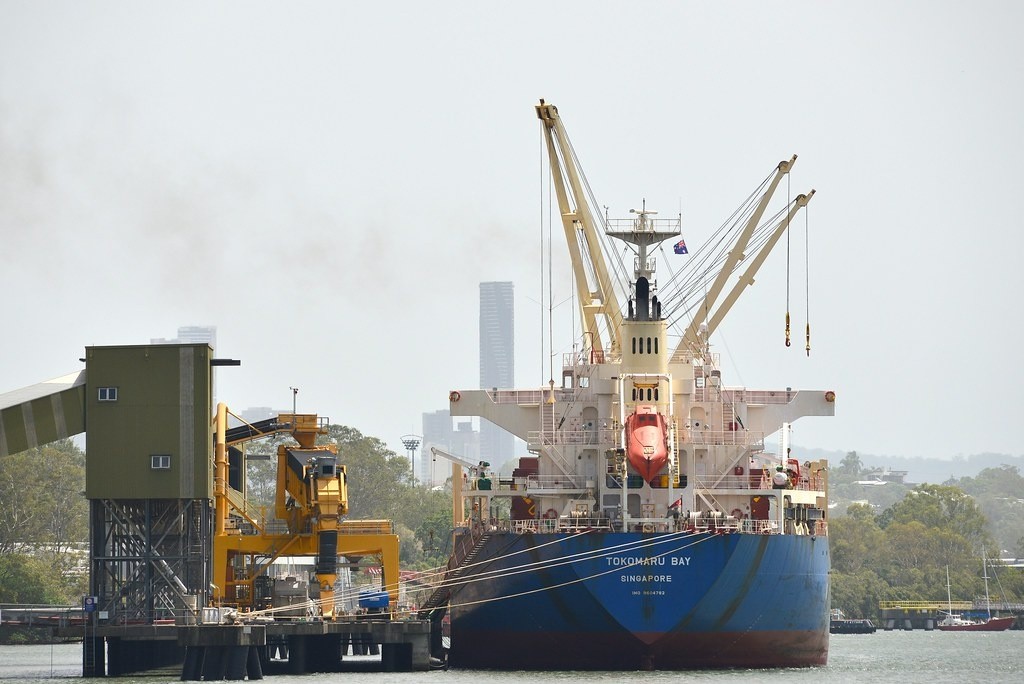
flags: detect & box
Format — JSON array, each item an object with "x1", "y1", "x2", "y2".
[
  {"x1": 411, "y1": 603, "x2": 415, "y2": 611},
  {"x1": 669, "y1": 498, "x2": 682, "y2": 509},
  {"x1": 673, "y1": 239, "x2": 688, "y2": 255}
]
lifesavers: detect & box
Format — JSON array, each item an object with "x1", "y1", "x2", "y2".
[
  {"x1": 546, "y1": 509, "x2": 557, "y2": 520},
  {"x1": 450, "y1": 392, "x2": 460, "y2": 401},
  {"x1": 825, "y1": 391, "x2": 835, "y2": 402},
  {"x1": 731, "y1": 509, "x2": 742, "y2": 520}
]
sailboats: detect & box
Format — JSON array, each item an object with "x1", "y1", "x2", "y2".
[{"x1": 938, "y1": 543, "x2": 1017, "y2": 631}]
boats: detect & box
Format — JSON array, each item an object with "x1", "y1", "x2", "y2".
[{"x1": 829, "y1": 620, "x2": 876, "y2": 634}]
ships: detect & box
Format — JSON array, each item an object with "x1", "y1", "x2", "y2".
[{"x1": 424, "y1": 100, "x2": 837, "y2": 670}]
